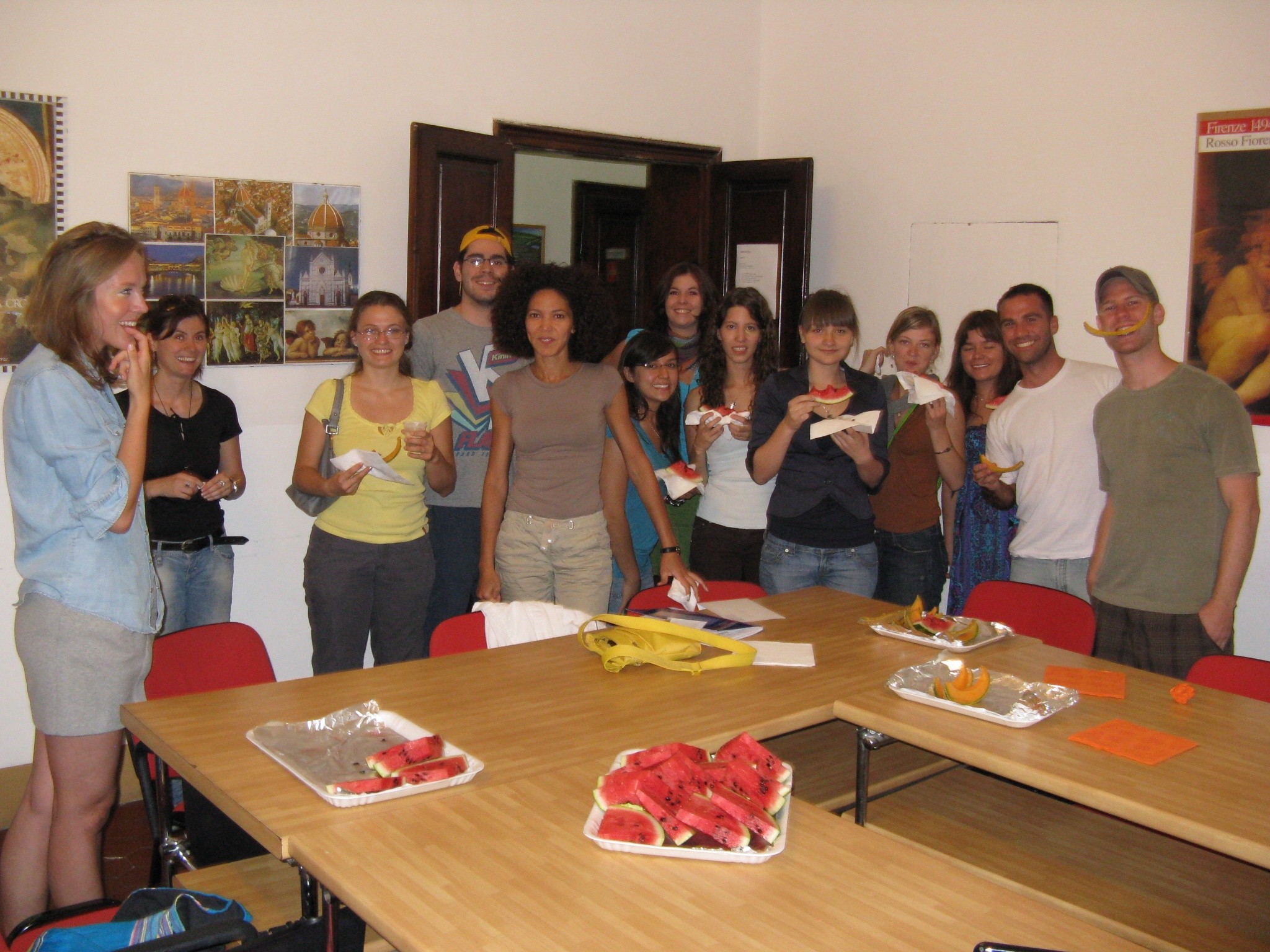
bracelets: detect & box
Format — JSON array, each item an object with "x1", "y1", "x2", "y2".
[{"x1": 934, "y1": 445, "x2": 955, "y2": 456}]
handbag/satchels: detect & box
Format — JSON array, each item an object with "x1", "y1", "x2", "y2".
[
  {"x1": 577, "y1": 613, "x2": 757, "y2": 673},
  {"x1": 285, "y1": 378, "x2": 344, "y2": 517}
]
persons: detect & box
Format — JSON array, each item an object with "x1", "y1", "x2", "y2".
[
  {"x1": 0, "y1": 220, "x2": 167, "y2": 943},
  {"x1": 1088, "y1": 264, "x2": 1261, "y2": 682},
  {"x1": 947, "y1": 310, "x2": 1015, "y2": 615},
  {"x1": 747, "y1": 283, "x2": 998, "y2": 459},
  {"x1": 746, "y1": 288, "x2": 891, "y2": 599},
  {"x1": 292, "y1": 291, "x2": 458, "y2": 677},
  {"x1": 597, "y1": 328, "x2": 685, "y2": 626},
  {"x1": 598, "y1": 265, "x2": 715, "y2": 608},
  {"x1": 973, "y1": 283, "x2": 1122, "y2": 629},
  {"x1": 686, "y1": 286, "x2": 781, "y2": 603},
  {"x1": 403, "y1": 225, "x2": 535, "y2": 665},
  {"x1": 114, "y1": 293, "x2": 247, "y2": 635},
  {"x1": 476, "y1": 264, "x2": 710, "y2": 634},
  {"x1": 857, "y1": 306, "x2": 967, "y2": 615}
]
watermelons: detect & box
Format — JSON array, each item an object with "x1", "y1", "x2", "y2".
[
  {"x1": 912, "y1": 613, "x2": 956, "y2": 636},
  {"x1": 591, "y1": 731, "x2": 794, "y2": 850},
  {"x1": 665, "y1": 460, "x2": 703, "y2": 482},
  {"x1": 985, "y1": 395, "x2": 1008, "y2": 409},
  {"x1": 325, "y1": 734, "x2": 471, "y2": 795},
  {"x1": 807, "y1": 385, "x2": 854, "y2": 404},
  {"x1": 700, "y1": 405, "x2": 734, "y2": 417}
]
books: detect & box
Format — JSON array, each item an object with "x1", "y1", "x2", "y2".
[{"x1": 627, "y1": 606, "x2": 762, "y2": 644}]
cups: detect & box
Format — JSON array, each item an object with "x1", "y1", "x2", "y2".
[{"x1": 403, "y1": 421, "x2": 428, "y2": 446}]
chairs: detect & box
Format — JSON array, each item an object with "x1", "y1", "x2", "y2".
[
  {"x1": 125, "y1": 623, "x2": 277, "y2": 888},
  {"x1": 963, "y1": 582, "x2": 1096, "y2": 656}
]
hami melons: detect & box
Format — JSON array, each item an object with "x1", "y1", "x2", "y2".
[{"x1": 931, "y1": 664, "x2": 991, "y2": 705}]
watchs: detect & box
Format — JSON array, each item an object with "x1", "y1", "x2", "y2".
[
  {"x1": 659, "y1": 545, "x2": 681, "y2": 555},
  {"x1": 227, "y1": 475, "x2": 238, "y2": 501}
]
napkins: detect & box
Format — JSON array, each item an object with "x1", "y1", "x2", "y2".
[
  {"x1": 667, "y1": 577, "x2": 698, "y2": 612},
  {"x1": 897, "y1": 371, "x2": 955, "y2": 418},
  {"x1": 810, "y1": 410, "x2": 881, "y2": 440},
  {"x1": 685, "y1": 410, "x2": 751, "y2": 426},
  {"x1": 329, "y1": 450, "x2": 412, "y2": 485},
  {"x1": 654, "y1": 464, "x2": 705, "y2": 500},
  {"x1": 733, "y1": 640, "x2": 815, "y2": 667}
]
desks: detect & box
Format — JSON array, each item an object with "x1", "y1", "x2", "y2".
[
  {"x1": 832, "y1": 644, "x2": 1270, "y2": 951},
  {"x1": 287, "y1": 754, "x2": 1153, "y2": 952},
  {"x1": 119, "y1": 586, "x2": 961, "y2": 952}
]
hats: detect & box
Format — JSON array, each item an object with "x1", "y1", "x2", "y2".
[
  {"x1": 1094, "y1": 266, "x2": 1160, "y2": 303},
  {"x1": 459, "y1": 225, "x2": 513, "y2": 257}
]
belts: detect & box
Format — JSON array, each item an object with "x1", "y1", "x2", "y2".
[{"x1": 150, "y1": 529, "x2": 247, "y2": 554}]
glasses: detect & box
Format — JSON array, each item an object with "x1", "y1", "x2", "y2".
[
  {"x1": 636, "y1": 361, "x2": 682, "y2": 371},
  {"x1": 356, "y1": 326, "x2": 411, "y2": 339},
  {"x1": 159, "y1": 293, "x2": 204, "y2": 311},
  {"x1": 170, "y1": 408, "x2": 188, "y2": 442},
  {"x1": 464, "y1": 256, "x2": 512, "y2": 268}
]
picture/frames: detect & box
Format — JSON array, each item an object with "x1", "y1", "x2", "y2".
[{"x1": 512, "y1": 224, "x2": 546, "y2": 265}]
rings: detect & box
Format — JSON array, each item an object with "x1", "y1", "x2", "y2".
[
  {"x1": 185, "y1": 480, "x2": 192, "y2": 487},
  {"x1": 218, "y1": 480, "x2": 225, "y2": 487}
]
